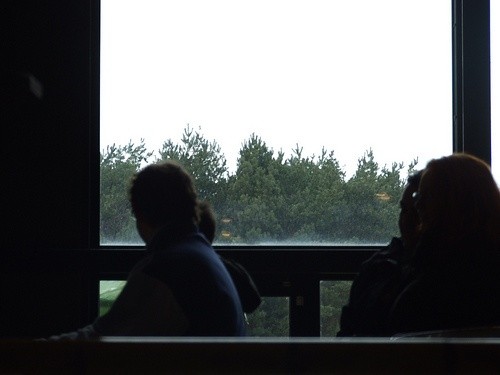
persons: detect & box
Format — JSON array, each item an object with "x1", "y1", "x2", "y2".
[
  {"x1": 337, "y1": 169, "x2": 427, "y2": 339},
  {"x1": 338, "y1": 152, "x2": 500, "y2": 339},
  {"x1": 195, "y1": 200, "x2": 261, "y2": 327},
  {"x1": 31, "y1": 161, "x2": 248, "y2": 341}
]
